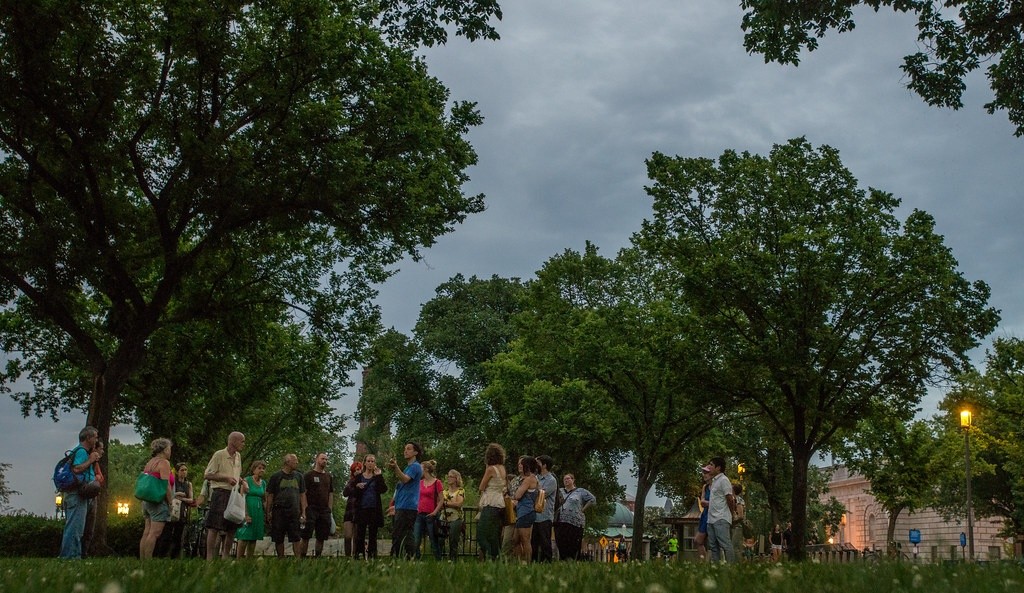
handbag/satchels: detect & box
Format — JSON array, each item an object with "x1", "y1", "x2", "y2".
[
  {"x1": 503, "y1": 497, "x2": 515, "y2": 525},
  {"x1": 166, "y1": 497, "x2": 182, "y2": 522},
  {"x1": 79, "y1": 480, "x2": 101, "y2": 500},
  {"x1": 223, "y1": 482, "x2": 246, "y2": 524},
  {"x1": 436, "y1": 520, "x2": 448, "y2": 537},
  {"x1": 134, "y1": 459, "x2": 169, "y2": 504}
]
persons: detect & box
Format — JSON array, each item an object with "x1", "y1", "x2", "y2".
[
  {"x1": 476, "y1": 443, "x2": 506, "y2": 560},
  {"x1": 158, "y1": 463, "x2": 193, "y2": 560},
  {"x1": 139, "y1": 438, "x2": 173, "y2": 559},
  {"x1": 192, "y1": 432, "x2": 249, "y2": 561},
  {"x1": 608, "y1": 538, "x2": 626, "y2": 562},
  {"x1": 769, "y1": 524, "x2": 783, "y2": 555},
  {"x1": 435, "y1": 469, "x2": 465, "y2": 559},
  {"x1": 266, "y1": 453, "x2": 305, "y2": 558},
  {"x1": 60, "y1": 426, "x2": 105, "y2": 560},
  {"x1": 387, "y1": 441, "x2": 422, "y2": 560},
  {"x1": 668, "y1": 534, "x2": 679, "y2": 559},
  {"x1": 342, "y1": 453, "x2": 388, "y2": 559},
  {"x1": 235, "y1": 460, "x2": 267, "y2": 559},
  {"x1": 413, "y1": 460, "x2": 441, "y2": 561},
  {"x1": 693, "y1": 457, "x2": 746, "y2": 566},
  {"x1": 301, "y1": 453, "x2": 334, "y2": 557},
  {"x1": 553, "y1": 473, "x2": 597, "y2": 561},
  {"x1": 783, "y1": 520, "x2": 792, "y2": 554},
  {"x1": 501, "y1": 454, "x2": 557, "y2": 564}
]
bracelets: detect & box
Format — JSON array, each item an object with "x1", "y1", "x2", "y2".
[{"x1": 241, "y1": 479, "x2": 245, "y2": 484}]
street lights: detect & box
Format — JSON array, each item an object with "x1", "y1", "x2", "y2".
[
  {"x1": 737, "y1": 462, "x2": 745, "y2": 499},
  {"x1": 960, "y1": 408, "x2": 974, "y2": 561},
  {"x1": 56, "y1": 492, "x2": 61, "y2": 519}
]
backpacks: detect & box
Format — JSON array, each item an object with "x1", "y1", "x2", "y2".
[
  {"x1": 54, "y1": 447, "x2": 88, "y2": 490},
  {"x1": 534, "y1": 490, "x2": 546, "y2": 513}
]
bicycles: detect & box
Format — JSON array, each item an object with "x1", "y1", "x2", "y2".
[{"x1": 863, "y1": 541, "x2": 909, "y2": 576}]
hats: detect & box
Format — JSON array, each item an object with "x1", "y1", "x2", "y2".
[{"x1": 702, "y1": 466, "x2": 711, "y2": 473}]
